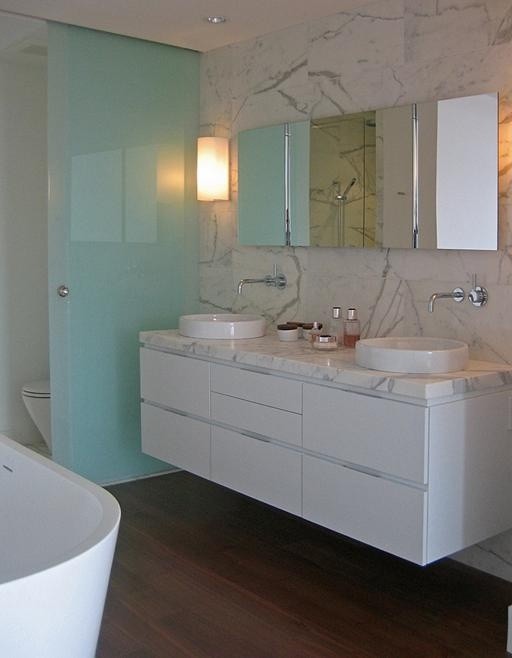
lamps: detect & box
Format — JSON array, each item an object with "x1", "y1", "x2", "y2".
[{"x1": 196, "y1": 137, "x2": 230, "y2": 201}]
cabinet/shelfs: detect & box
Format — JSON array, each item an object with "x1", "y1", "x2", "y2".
[{"x1": 140, "y1": 345, "x2": 512, "y2": 567}]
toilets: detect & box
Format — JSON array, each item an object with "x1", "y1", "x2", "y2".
[{"x1": 21, "y1": 379, "x2": 53, "y2": 454}]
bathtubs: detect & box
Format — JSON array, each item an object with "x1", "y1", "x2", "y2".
[{"x1": 0, "y1": 425, "x2": 122, "y2": 658}]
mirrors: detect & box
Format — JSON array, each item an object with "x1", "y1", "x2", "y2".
[{"x1": 237, "y1": 90, "x2": 499, "y2": 252}]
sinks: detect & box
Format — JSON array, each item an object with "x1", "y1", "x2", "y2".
[
  {"x1": 355, "y1": 336, "x2": 469, "y2": 376},
  {"x1": 179, "y1": 313, "x2": 266, "y2": 340}
]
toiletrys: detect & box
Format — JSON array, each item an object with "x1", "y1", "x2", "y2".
[{"x1": 276, "y1": 306, "x2": 360, "y2": 354}]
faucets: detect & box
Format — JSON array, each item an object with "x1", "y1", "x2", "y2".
[
  {"x1": 237, "y1": 273, "x2": 273, "y2": 295},
  {"x1": 427, "y1": 287, "x2": 465, "y2": 313}
]
